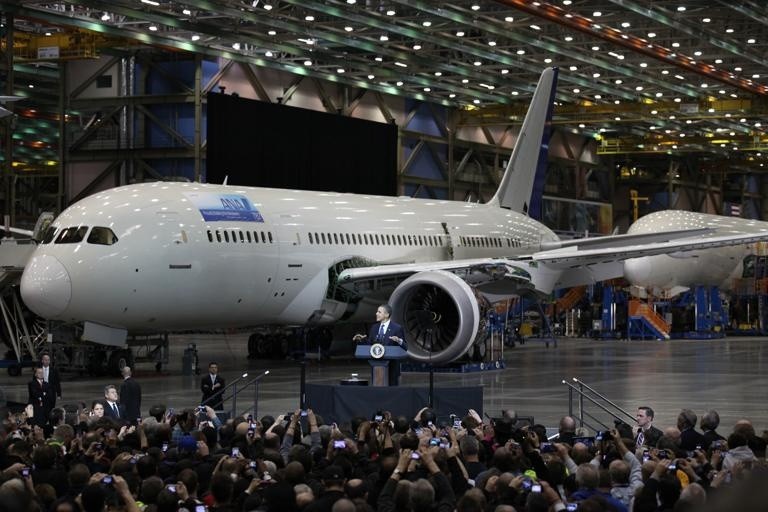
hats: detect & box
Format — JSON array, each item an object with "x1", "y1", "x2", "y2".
[
  {"x1": 323, "y1": 465, "x2": 344, "y2": 479},
  {"x1": 181, "y1": 436, "x2": 199, "y2": 448}
]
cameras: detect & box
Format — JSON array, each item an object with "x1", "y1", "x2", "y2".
[{"x1": 21, "y1": 405, "x2": 725, "y2": 512}]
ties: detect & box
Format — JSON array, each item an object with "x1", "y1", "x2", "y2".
[
  {"x1": 638, "y1": 433, "x2": 643, "y2": 446},
  {"x1": 113, "y1": 404, "x2": 118, "y2": 416},
  {"x1": 380, "y1": 325, "x2": 384, "y2": 342}
]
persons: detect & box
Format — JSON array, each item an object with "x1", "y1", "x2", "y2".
[
  {"x1": 199, "y1": 362, "x2": 225, "y2": 410},
  {"x1": 0, "y1": 400, "x2": 768, "y2": 512},
  {"x1": 351, "y1": 301, "x2": 407, "y2": 386},
  {"x1": 27, "y1": 367, "x2": 55, "y2": 424},
  {"x1": 119, "y1": 366, "x2": 142, "y2": 422},
  {"x1": 38, "y1": 353, "x2": 63, "y2": 406},
  {"x1": 100, "y1": 384, "x2": 130, "y2": 426}
]
